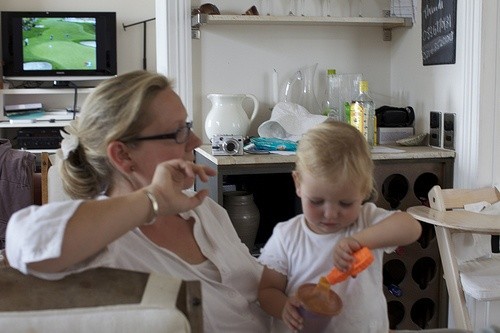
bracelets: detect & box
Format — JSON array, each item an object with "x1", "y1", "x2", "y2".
[{"x1": 139, "y1": 187, "x2": 159, "y2": 226}]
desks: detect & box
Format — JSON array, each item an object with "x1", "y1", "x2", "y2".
[{"x1": 0, "y1": 264, "x2": 195, "y2": 333}]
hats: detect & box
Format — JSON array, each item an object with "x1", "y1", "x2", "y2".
[{"x1": 257, "y1": 101, "x2": 328, "y2": 142}]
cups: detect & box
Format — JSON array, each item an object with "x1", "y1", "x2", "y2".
[{"x1": 292, "y1": 283, "x2": 344, "y2": 332}]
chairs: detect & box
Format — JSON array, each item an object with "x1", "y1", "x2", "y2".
[
  {"x1": 0, "y1": 139, "x2": 51, "y2": 206},
  {"x1": 406, "y1": 185, "x2": 500, "y2": 333}
]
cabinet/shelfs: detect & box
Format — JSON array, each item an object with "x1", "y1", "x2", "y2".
[
  {"x1": 0, "y1": 77, "x2": 116, "y2": 152},
  {"x1": 194, "y1": 144, "x2": 455, "y2": 329}
]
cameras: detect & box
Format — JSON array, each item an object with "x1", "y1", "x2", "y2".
[{"x1": 211, "y1": 135, "x2": 244, "y2": 156}]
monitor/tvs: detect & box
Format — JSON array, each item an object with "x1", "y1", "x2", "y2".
[{"x1": 1, "y1": 11, "x2": 117, "y2": 76}]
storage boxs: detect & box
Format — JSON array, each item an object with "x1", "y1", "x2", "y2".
[{"x1": 377, "y1": 127, "x2": 414, "y2": 146}]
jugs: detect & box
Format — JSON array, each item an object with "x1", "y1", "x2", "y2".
[
  {"x1": 204, "y1": 93, "x2": 260, "y2": 140},
  {"x1": 283, "y1": 63, "x2": 325, "y2": 115}
]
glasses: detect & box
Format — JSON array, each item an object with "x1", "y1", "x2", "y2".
[{"x1": 121, "y1": 121, "x2": 193, "y2": 144}]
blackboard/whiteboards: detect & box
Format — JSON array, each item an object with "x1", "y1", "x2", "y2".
[{"x1": 421, "y1": 0, "x2": 458, "y2": 66}]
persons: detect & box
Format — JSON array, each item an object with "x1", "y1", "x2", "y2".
[
  {"x1": 256, "y1": 121, "x2": 422, "y2": 333},
  {"x1": 5, "y1": 71, "x2": 294, "y2": 333}
]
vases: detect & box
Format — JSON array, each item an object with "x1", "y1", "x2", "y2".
[{"x1": 224, "y1": 192, "x2": 260, "y2": 253}]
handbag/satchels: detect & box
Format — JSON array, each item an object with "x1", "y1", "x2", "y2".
[{"x1": 376, "y1": 105, "x2": 415, "y2": 127}]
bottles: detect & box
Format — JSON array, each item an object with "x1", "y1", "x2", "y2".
[
  {"x1": 223, "y1": 191, "x2": 261, "y2": 250},
  {"x1": 321, "y1": 247, "x2": 374, "y2": 287},
  {"x1": 323, "y1": 69, "x2": 344, "y2": 115},
  {"x1": 351, "y1": 81, "x2": 374, "y2": 150}
]
